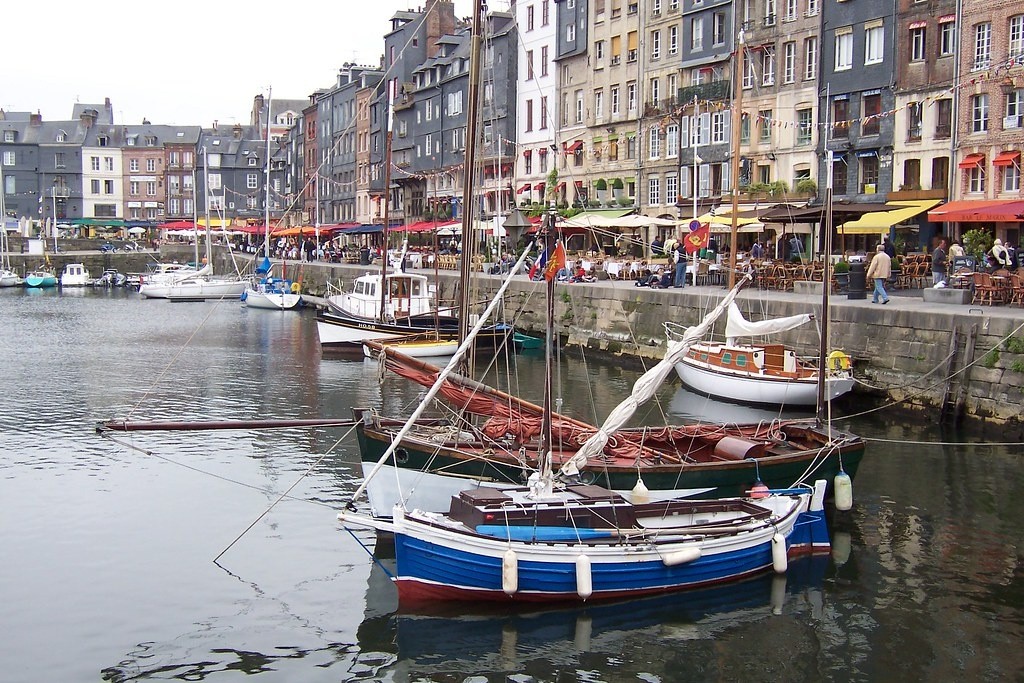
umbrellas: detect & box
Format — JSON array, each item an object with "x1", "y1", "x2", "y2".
[
  {"x1": 553, "y1": 204, "x2": 922, "y2": 261},
  {"x1": 387, "y1": 220, "x2": 495, "y2": 254}
]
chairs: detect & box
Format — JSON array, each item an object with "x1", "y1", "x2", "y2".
[
  {"x1": 339, "y1": 251, "x2": 486, "y2": 272},
  {"x1": 578, "y1": 253, "x2": 1024, "y2": 308}
]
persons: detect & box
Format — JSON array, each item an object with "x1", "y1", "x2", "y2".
[
  {"x1": 30, "y1": 227, "x2": 1016, "y2": 289},
  {"x1": 866, "y1": 244, "x2": 891, "y2": 304}
]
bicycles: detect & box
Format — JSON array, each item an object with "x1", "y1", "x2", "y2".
[{"x1": 49, "y1": 244, "x2": 67, "y2": 255}]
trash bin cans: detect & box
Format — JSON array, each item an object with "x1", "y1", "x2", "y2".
[
  {"x1": 847, "y1": 263, "x2": 867, "y2": 299},
  {"x1": 360, "y1": 248, "x2": 369, "y2": 265}
]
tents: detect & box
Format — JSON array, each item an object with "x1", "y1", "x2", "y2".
[{"x1": 157, "y1": 219, "x2": 384, "y2": 251}]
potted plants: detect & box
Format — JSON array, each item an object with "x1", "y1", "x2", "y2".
[
  {"x1": 752, "y1": 182, "x2": 770, "y2": 192},
  {"x1": 520, "y1": 202, "x2": 527, "y2": 208},
  {"x1": 588, "y1": 201, "x2": 599, "y2": 208},
  {"x1": 796, "y1": 179, "x2": 816, "y2": 194},
  {"x1": 611, "y1": 200, "x2": 617, "y2": 208},
  {"x1": 613, "y1": 178, "x2": 625, "y2": 203},
  {"x1": 532, "y1": 202, "x2": 538, "y2": 209},
  {"x1": 885, "y1": 256, "x2": 902, "y2": 291},
  {"x1": 597, "y1": 178, "x2": 608, "y2": 203},
  {"x1": 517, "y1": 249, "x2": 527, "y2": 272},
  {"x1": 624, "y1": 198, "x2": 631, "y2": 207},
  {"x1": 833, "y1": 261, "x2": 850, "y2": 295},
  {"x1": 770, "y1": 180, "x2": 789, "y2": 195},
  {"x1": 618, "y1": 199, "x2": 624, "y2": 208}
]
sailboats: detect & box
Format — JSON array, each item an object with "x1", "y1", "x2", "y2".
[{"x1": 0, "y1": 1, "x2": 860, "y2": 621}]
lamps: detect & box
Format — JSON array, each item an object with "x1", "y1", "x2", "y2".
[
  {"x1": 659, "y1": 129, "x2": 668, "y2": 140},
  {"x1": 999, "y1": 61, "x2": 1016, "y2": 95}
]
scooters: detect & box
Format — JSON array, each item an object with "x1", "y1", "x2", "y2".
[
  {"x1": 100, "y1": 240, "x2": 118, "y2": 254},
  {"x1": 122, "y1": 239, "x2": 144, "y2": 254}
]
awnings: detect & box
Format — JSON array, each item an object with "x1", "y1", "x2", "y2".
[
  {"x1": 46, "y1": 218, "x2": 231, "y2": 229},
  {"x1": 556, "y1": 209, "x2": 631, "y2": 219},
  {"x1": 927, "y1": 198, "x2": 1023, "y2": 221},
  {"x1": 836, "y1": 198, "x2": 943, "y2": 233},
  {"x1": 680, "y1": 203, "x2": 805, "y2": 233}
]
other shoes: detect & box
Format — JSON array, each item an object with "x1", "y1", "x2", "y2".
[
  {"x1": 882, "y1": 299, "x2": 889, "y2": 304},
  {"x1": 872, "y1": 300, "x2": 878, "y2": 303}
]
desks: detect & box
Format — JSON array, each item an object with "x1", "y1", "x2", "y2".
[
  {"x1": 951, "y1": 275, "x2": 1013, "y2": 304},
  {"x1": 709, "y1": 264, "x2": 721, "y2": 285},
  {"x1": 581, "y1": 262, "x2": 598, "y2": 270},
  {"x1": 631, "y1": 262, "x2": 642, "y2": 279},
  {"x1": 603, "y1": 261, "x2": 611, "y2": 273},
  {"x1": 566, "y1": 261, "x2": 577, "y2": 275},
  {"x1": 685, "y1": 266, "x2": 693, "y2": 287},
  {"x1": 647, "y1": 263, "x2": 664, "y2": 274},
  {"x1": 608, "y1": 263, "x2": 626, "y2": 280}
]
